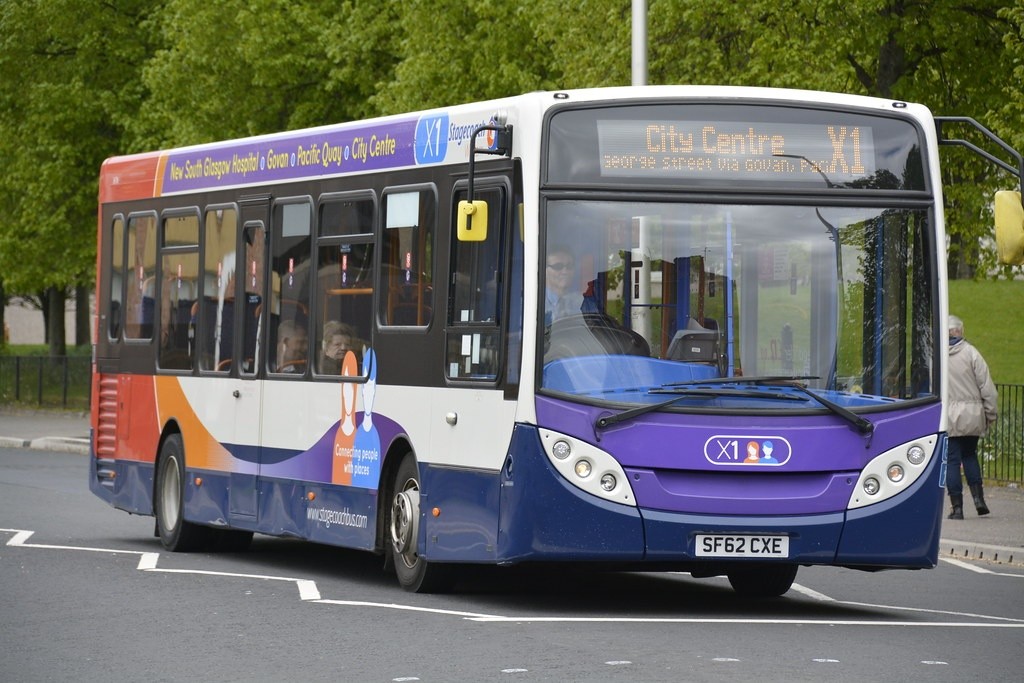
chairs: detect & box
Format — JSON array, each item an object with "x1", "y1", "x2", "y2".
[{"x1": 138, "y1": 277, "x2": 433, "y2": 374}]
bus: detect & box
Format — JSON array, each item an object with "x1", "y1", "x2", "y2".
[{"x1": 88, "y1": 85, "x2": 1024, "y2": 595}]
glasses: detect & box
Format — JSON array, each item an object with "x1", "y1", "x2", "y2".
[{"x1": 547, "y1": 262, "x2": 576, "y2": 272}]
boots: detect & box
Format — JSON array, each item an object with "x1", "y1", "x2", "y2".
[
  {"x1": 949, "y1": 494, "x2": 964, "y2": 520},
  {"x1": 969, "y1": 484, "x2": 990, "y2": 515}
]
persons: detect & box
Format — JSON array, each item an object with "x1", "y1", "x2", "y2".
[
  {"x1": 930, "y1": 316, "x2": 998, "y2": 519},
  {"x1": 322, "y1": 322, "x2": 363, "y2": 375},
  {"x1": 545, "y1": 245, "x2": 599, "y2": 325},
  {"x1": 277, "y1": 320, "x2": 307, "y2": 372}
]
